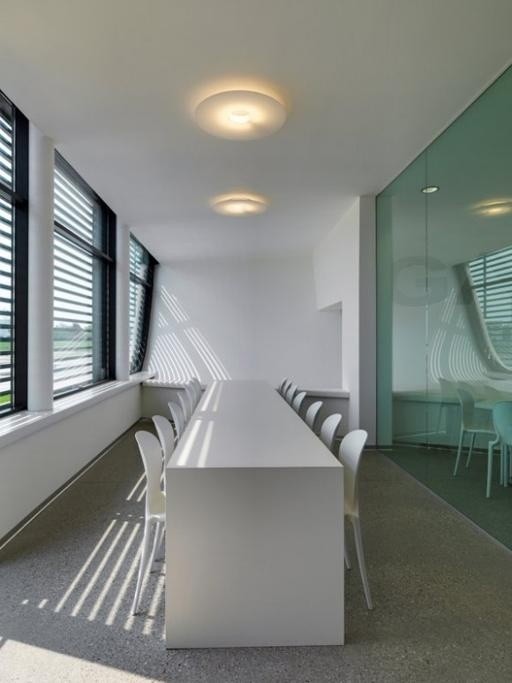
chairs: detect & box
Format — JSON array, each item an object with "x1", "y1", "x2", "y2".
[
  {"x1": 278, "y1": 379, "x2": 375, "y2": 614},
  {"x1": 435, "y1": 378, "x2": 458, "y2": 434},
  {"x1": 132, "y1": 378, "x2": 203, "y2": 614},
  {"x1": 451, "y1": 387, "x2": 504, "y2": 485},
  {"x1": 486, "y1": 400, "x2": 512, "y2": 498}
]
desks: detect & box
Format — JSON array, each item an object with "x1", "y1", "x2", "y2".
[
  {"x1": 474, "y1": 399, "x2": 504, "y2": 411},
  {"x1": 166, "y1": 380, "x2": 346, "y2": 650}
]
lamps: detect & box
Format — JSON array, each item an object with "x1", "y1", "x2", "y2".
[
  {"x1": 194, "y1": 89, "x2": 287, "y2": 141},
  {"x1": 213, "y1": 194, "x2": 267, "y2": 216},
  {"x1": 472, "y1": 199, "x2": 512, "y2": 216}
]
